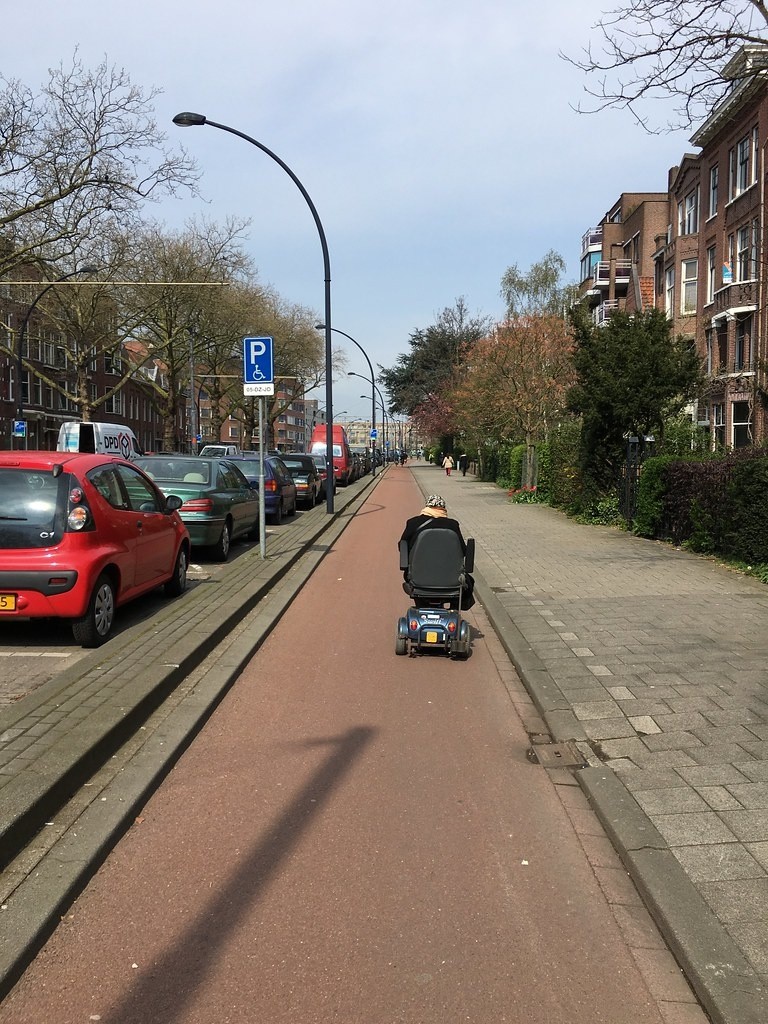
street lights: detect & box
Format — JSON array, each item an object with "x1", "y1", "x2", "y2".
[
  {"x1": 348, "y1": 372, "x2": 385, "y2": 464},
  {"x1": 15, "y1": 265, "x2": 97, "y2": 422},
  {"x1": 173, "y1": 112, "x2": 335, "y2": 511},
  {"x1": 314, "y1": 325, "x2": 375, "y2": 475},
  {"x1": 360, "y1": 395, "x2": 389, "y2": 461},
  {"x1": 376, "y1": 407, "x2": 396, "y2": 451},
  {"x1": 311, "y1": 405, "x2": 333, "y2": 435},
  {"x1": 330, "y1": 411, "x2": 347, "y2": 428}
]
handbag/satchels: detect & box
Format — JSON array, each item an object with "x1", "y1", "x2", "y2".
[{"x1": 451, "y1": 462, "x2": 453, "y2": 465}]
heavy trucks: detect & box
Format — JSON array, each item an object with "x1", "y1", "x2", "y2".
[{"x1": 307, "y1": 426, "x2": 365, "y2": 486}]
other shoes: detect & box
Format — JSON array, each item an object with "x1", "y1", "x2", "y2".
[
  {"x1": 463, "y1": 475, "x2": 465, "y2": 476},
  {"x1": 447, "y1": 474, "x2": 450, "y2": 476}
]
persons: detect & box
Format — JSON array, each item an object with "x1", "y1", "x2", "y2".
[
  {"x1": 398, "y1": 495, "x2": 476, "y2": 611},
  {"x1": 439, "y1": 452, "x2": 444, "y2": 468},
  {"x1": 417, "y1": 452, "x2": 419, "y2": 460},
  {"x1": 410, "y1": 452, "x2": 413, "y2": 459},
  {"x1": 460, "y1": 453, "x2": 467, "y2": 476},
  {"x1": 442, "y1": 453, "x2": 454, "y2": 476},
  {"x1": 429, "y1": 453, "x2": 434, "y2": 464},
  {"x1": 393, "y1": 452, "x2": 408, "y2": 467}
]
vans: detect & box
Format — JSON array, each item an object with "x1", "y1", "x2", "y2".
[
  {"x1": 57, "y1": 422, "x2": 144, "y2": 460},
  {"x1": 268, "y1": 450, "x2": 282, "y2": 456},
  {"x1": 198, "y1": 445, "x2": 237, "y2": 455}
]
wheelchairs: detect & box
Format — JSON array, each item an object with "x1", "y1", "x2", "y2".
[{"x1": 396, "y1": 529, "x2": 476, "y2": 659}]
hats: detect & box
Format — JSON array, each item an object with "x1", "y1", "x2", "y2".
[{"x1": 425, "y1": 494, "x2": 445, "y2": 508}]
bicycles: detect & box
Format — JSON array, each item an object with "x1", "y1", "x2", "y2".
[{"x1": 394, "y1": 456, "x2": 408, "y2": 467}]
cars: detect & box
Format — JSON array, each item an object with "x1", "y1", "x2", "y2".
[
  {"x1": 158, "y1": 452, "x2": 174, "y2": 455},
  {"x1": 349, "y1": 446, "x2": 424, "y2": 467},
  {"x1": 144, "y1": 451, "x2": 156, "y2": 455},
  {"x1": 280, "y1": 455, "x2": 322, "y2": 509},
  {"x1": 240, "y1": 451, "x2": 259, "y2": 455},
  {"x1": 0, "y1": 450, "x2": 190, "y2": 646},
  {"x1": 131, "y1": 456, "x2": 259, "y2": 562},
  {"x1": 220, "y1": 457, "x2": 297, "y2": 525},
  {"x1": 309, "y1": 455, "x2": 338, "y2": 499}
]
